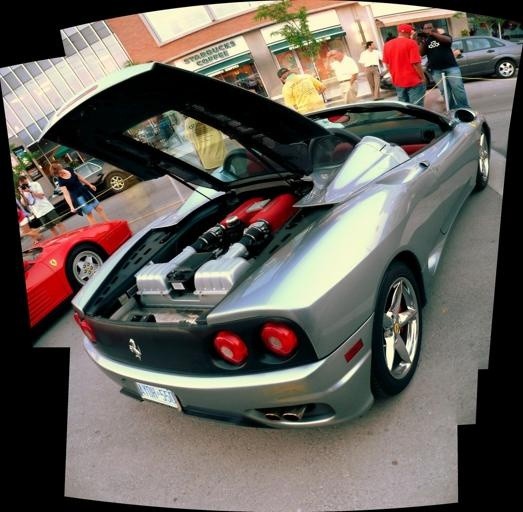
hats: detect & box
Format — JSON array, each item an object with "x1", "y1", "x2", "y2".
[
  {"x1": 397, "y1": 24, "x2": 416, "y2": 36},
  {"x1": 276, "y1": 68, "x2": 288, "y2": 78}
]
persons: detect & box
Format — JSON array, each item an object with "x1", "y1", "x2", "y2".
[
  {"x1": 17, "y1": 175, "x2": 67, "y2": 237},
  {"x1": 415, "y1": 21, "x2": 471, "y2": 115},
  {"x1": 436, "y1": 28, "x2": 461, "y2": 59},
  {"x1": 357, "y1": 40, "x2": 385, "y2": 102},
  {"x1": 384, "y1": 29, "x2": 395, "y2": 44},
  {"x1": 325, "y1": 48, "x2": 360, "y2": 105},
  {"x1": 15, "y1": 204, "x2": 43, "y2": 245},
  {"x1": 276, "y1": 67, "x2": 344, "y2": 130},
  {"x1": 48, "y1": 162, "x2": 109, "y2": 224},
  {"x1": 381, "y1": 23, "x2": 427, "y2": 108},
  {"x1": 184, "y1": 117, "x2": 227, "y2": 174}
]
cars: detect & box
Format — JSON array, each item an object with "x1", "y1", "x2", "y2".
[
  {"x1": 39, "y1": 61, "x2": 490, "y2": 430},
  {"x1": 21, "y1": 220, "x2": 133, "y2": 328},
  {"x1": 380, "y1": 35, "x2": 523, "y2": 91},
  {"x1": 44, "y1": 155, "x2": 138, "y2": 201}
]
